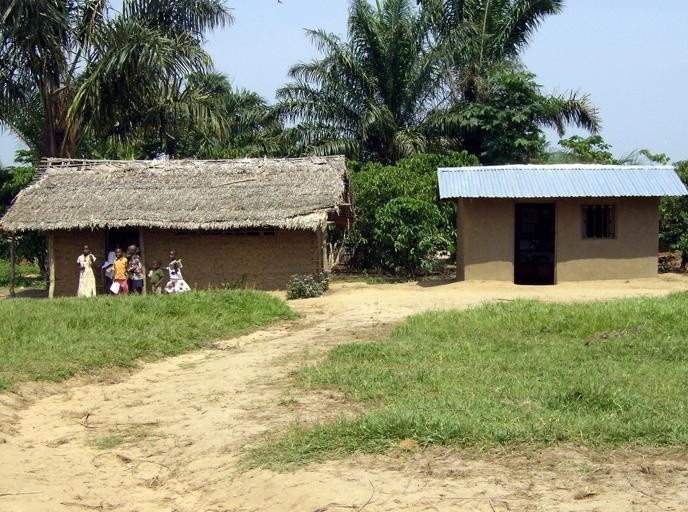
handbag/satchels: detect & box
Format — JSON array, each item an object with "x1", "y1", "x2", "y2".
[{"x1": 110, "y1": 282, "x2": 121, "y2": 294}]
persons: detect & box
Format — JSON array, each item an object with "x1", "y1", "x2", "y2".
[
  {"x1": 76, "y1": 244, "x2": 97, "y2": 298},
  {"x1": 101, "y1": 242, "x2": 191, "y2": 296}
]
